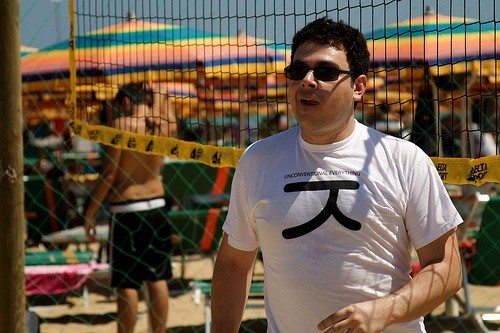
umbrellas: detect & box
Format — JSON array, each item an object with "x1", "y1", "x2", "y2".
[{"x1": 16, "y1": 6, "x2": 500, "y2": 135}]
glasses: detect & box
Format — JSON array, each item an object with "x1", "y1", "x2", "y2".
[{"x1": 284, "y1": 63, "x2": 356, "y2": 82}]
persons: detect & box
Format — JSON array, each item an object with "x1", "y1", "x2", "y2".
[
  {"x1": 84, "y1": 82, "x2": 500, "y2": 333},
  {"x1": 210, "y1": 16, "x2": 463, "y2": 333}
]
